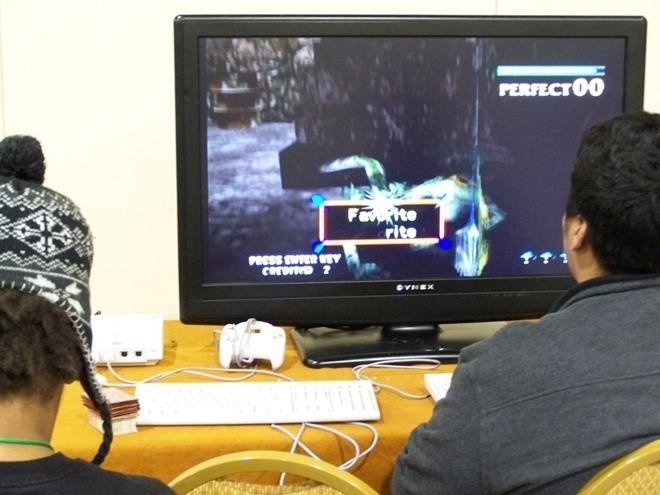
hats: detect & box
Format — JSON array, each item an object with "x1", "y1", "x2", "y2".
[{"x1": 0, "y1": 135, "x2": 112, "y2": 465}]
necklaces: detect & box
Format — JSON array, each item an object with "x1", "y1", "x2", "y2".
[{"x1": 1, "y1": 436, "x2": 54, "y2": 451}]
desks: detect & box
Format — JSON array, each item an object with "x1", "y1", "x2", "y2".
[{"x1": 50, "y1": 320, "x2": 460, "y2": 488}]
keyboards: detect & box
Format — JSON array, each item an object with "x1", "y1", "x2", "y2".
[{"x1": 134, "y1": 380, "x2": 381, "y2": 427}]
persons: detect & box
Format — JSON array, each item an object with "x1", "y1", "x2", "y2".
[
  {"x1": 0, "y1": 284, "x2": 177, "y2": 495},
  {"x1": 390, "y1": 108, "x2": 660, "y2": 495},
  {"x1": 0, "y1": 135, "x2": 95, "y2": 360}
]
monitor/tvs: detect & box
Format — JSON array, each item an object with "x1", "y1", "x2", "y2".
[{"x1": 162, "y1": 13, "x2": 648, "y2": 365}]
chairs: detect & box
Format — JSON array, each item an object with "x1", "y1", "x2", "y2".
[{"x1": 158, "y1": 451, "x2": 384, "y2": 495}]
